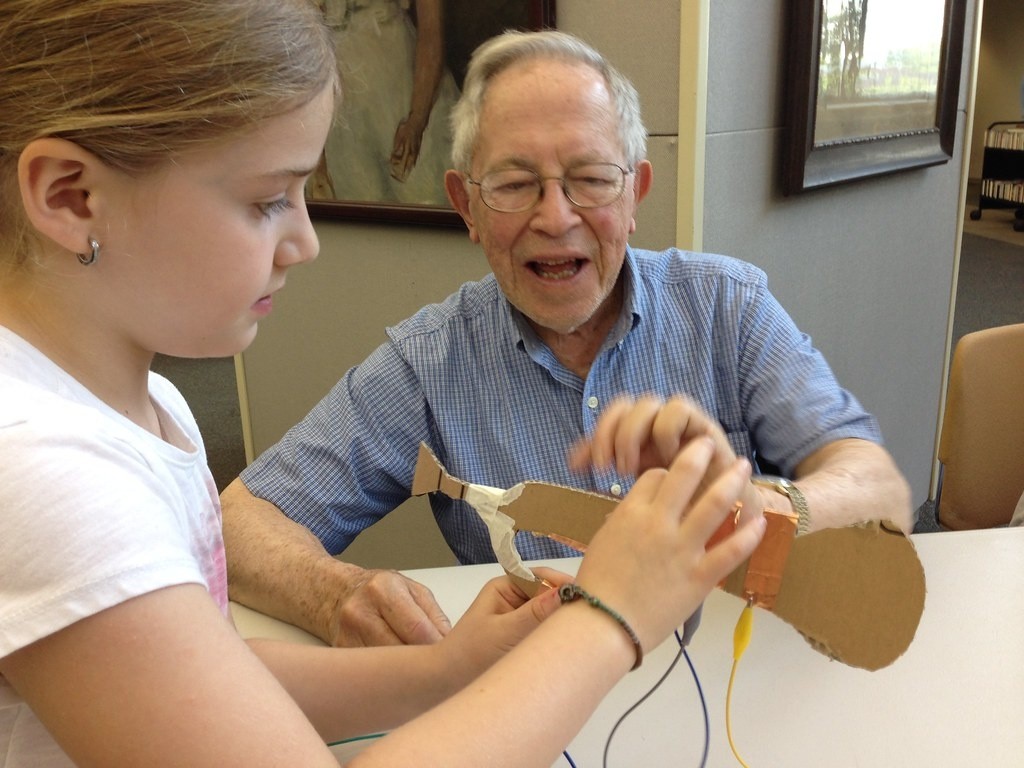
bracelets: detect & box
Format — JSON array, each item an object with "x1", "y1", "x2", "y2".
[{"x1": 559, "y1": 584, "x2": 642, "y2": 671}]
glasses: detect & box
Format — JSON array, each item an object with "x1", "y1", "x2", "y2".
[{"x1": 466, "y1": 163, "x2": 636, "y2": 213}]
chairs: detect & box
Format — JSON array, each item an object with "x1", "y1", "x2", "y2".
[{"x1": 934, "y1": 324, "x2": 1024, "y2": 529}]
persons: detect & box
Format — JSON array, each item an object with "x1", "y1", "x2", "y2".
[
  {"x1": 0, "y1": 0, "x2": 766, "y2": 765},
  {"x1": 218, "y1": 29, "x2": 912, "y2": 648}
]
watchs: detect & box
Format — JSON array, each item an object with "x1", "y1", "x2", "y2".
[{"x1": 751, "y1": 475, "x2": 808, "y2": 538}]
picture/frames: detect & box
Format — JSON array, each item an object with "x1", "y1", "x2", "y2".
[
  {"x1": 779, "y1": 0, "x2": 969, "y2": 197},
  {"x1": 291, "y1": 1, "x2": 557, "y2": 233}
]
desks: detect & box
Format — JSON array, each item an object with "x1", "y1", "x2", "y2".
[{"x1": 228, "y1": 526, "x2": 1024, "y2": 768}]
